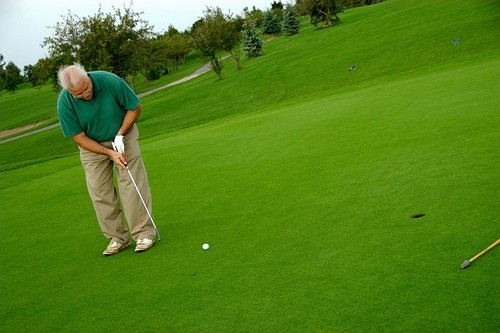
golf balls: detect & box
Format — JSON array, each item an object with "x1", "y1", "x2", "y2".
[{"x1": 202, "y1": 244, "x2": 209, "y2": 250}]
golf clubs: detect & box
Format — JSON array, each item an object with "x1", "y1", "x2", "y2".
[{"x1": 112, "y1": 141, "x2": 160, "y2": 241}]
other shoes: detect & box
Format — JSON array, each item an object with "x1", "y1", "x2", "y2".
[
  {"x1": 102, "y1": 240, "x2": 132, "y2": 255},
  {"x1": 134, "y1": 238, "x2": 156, "y2": 252}
]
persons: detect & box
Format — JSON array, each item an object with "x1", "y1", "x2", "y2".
[{"x1": 57, "y1": 64, "x2": 156, "y2": 256}]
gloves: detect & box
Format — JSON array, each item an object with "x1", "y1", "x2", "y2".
[{"x1": 114, "y1": 135, "x2": 125, "y2": 155}]
